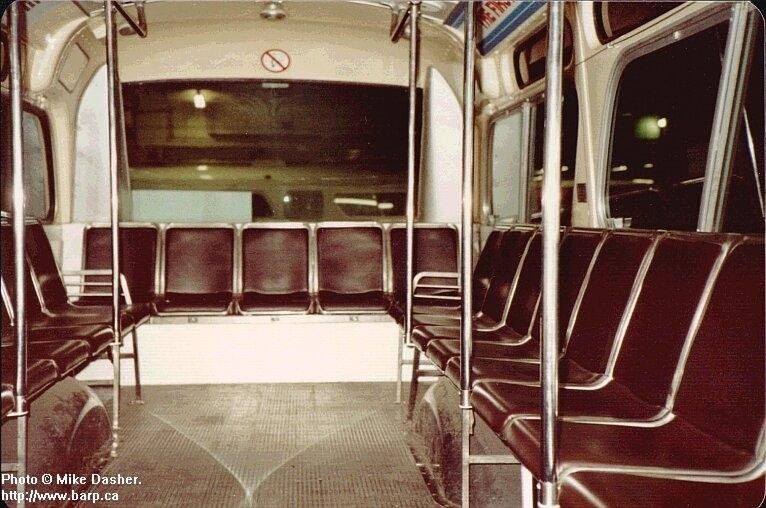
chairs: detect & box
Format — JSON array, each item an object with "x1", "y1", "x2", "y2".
[
  {"x1": 156, "y1": 220, "x2": 391, "y2": 318},
  {"x1": 0, "y1": 209, "x2": 160, "y2": 507},
  {"x1": 388, "y1": 221, "x2": 766, "y2": 508}
]
